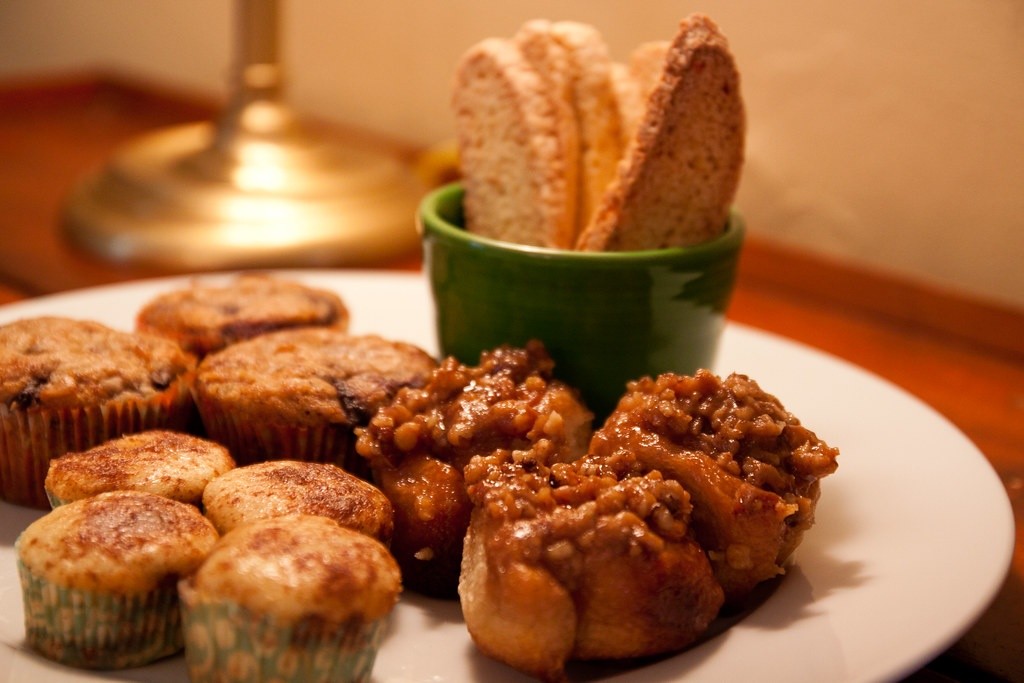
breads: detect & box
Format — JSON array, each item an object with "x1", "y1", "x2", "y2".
[
  {"x1": 452, "y1": 13, "x2": 749, "y2": 251},
  {"x1": 354, "y1": 346, "x2": 838, "y2": 672}
]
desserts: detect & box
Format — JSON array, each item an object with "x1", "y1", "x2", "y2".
[{"x1": 0, "y1": 257, "x2": 439, "y2": 683}]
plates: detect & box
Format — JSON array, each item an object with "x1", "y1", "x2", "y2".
[{"x1": 0, "y1": 270, "x2": 1017, "y2": 683}]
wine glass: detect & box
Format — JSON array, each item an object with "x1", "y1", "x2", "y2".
[{"x1": 59, "y1": 0, "x2": 442, "y2": 268}]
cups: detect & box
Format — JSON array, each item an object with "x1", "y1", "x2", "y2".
[{"x1": 418, "y1": 181, "x2": 746, "y2": 428}]
